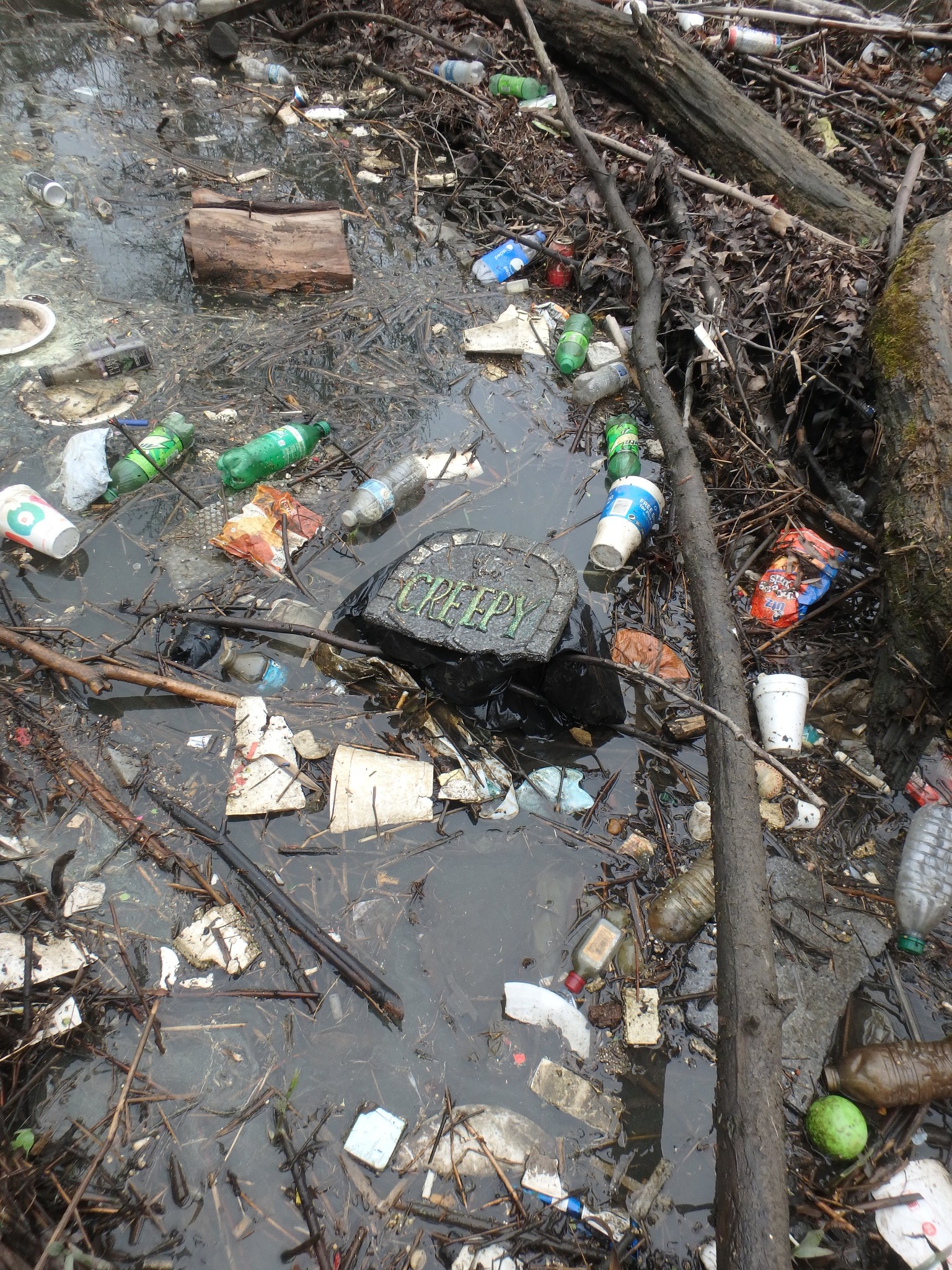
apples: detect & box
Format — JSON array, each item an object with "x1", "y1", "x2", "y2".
[{"x1": 806, "y1": 1095, "x2": 868, "y2": 1160}]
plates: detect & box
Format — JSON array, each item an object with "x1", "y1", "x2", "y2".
[
  {"x1": 19, "y1": 375, "x2": 140, "y2": 426},
  {"x1": 0, "y1": 298, "x2": 55, "y2": 355}
]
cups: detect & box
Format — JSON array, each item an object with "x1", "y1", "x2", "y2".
[
  {"x1": 688, "y1": 801, "x2": 712, "y2": 841},
  {"x1": 753, "y1": 673, "x2": 809, "y2": 760},
  {"x1": 590, "y1": 475, "x2": 666, "y2": 571},
  {"x1": 779, "y1": 795, "x2": 821, "y2": 830},
  {"x1": 676, "y1": 11, "x2": 704, "y2": 31},
  {"x1": 0, "y1": 484, "x2": 81, "y2": 559},
  {"x1": 623, "y1": 0, "x2": 647, "y2": 18}
]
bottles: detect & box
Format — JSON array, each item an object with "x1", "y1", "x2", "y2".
[
  {"x1": 895, "y1": 803, "x2": 952, "y2": 955},
  {"x1": 564, "y1": 916, "x2": 625, "y2": 994},
  {"x1": 39, "y1": 329, "x2": 154, "y2": 388},
  {"x1": 98, "y1": 412, "x2": 194, "y2": 504},
  {"x1": 218, "y1": 649, "x2": 289, "y2": 692},
  {"x1": 823, "y1": 1040, "x2": 952, "y2": 1109},
  {"x1": 489, "y1": 74, "x2": 547, "y2": 100},
  {"x1": 341, "y1": 454, "x2": 427, "y2": 527},
  {"x1": 470, "y1": 231, "x2": 665, "y2": 479},
  {"x1": 434, "y1": 60, "x2": 486, "y2": 85},
  {"x1": 215, "y1": 421, "x2": 330, "y2": 489},
  {"x1": 117, "y1": 0, "x2": 297, "y2": 86},
  {"x1": 647, "y1": 846, "x2": 715, "y2": 943}
]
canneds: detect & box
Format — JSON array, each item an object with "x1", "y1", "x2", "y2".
[
  {"x1": 288, "y1": 86, "x2": 310, "y2": 108},
  {"x1": 547, "y1": 236, "x2": 575, "y2": 289},
  {"x1": 22, "y1": 172, "x2": 67, "y2": 207},
  {"x1": 92, "y1": 197, "x2": 112, "y2": 215},
  {"x1": 720, "y1": 26, "x2": 781, "y2": 57}
]
